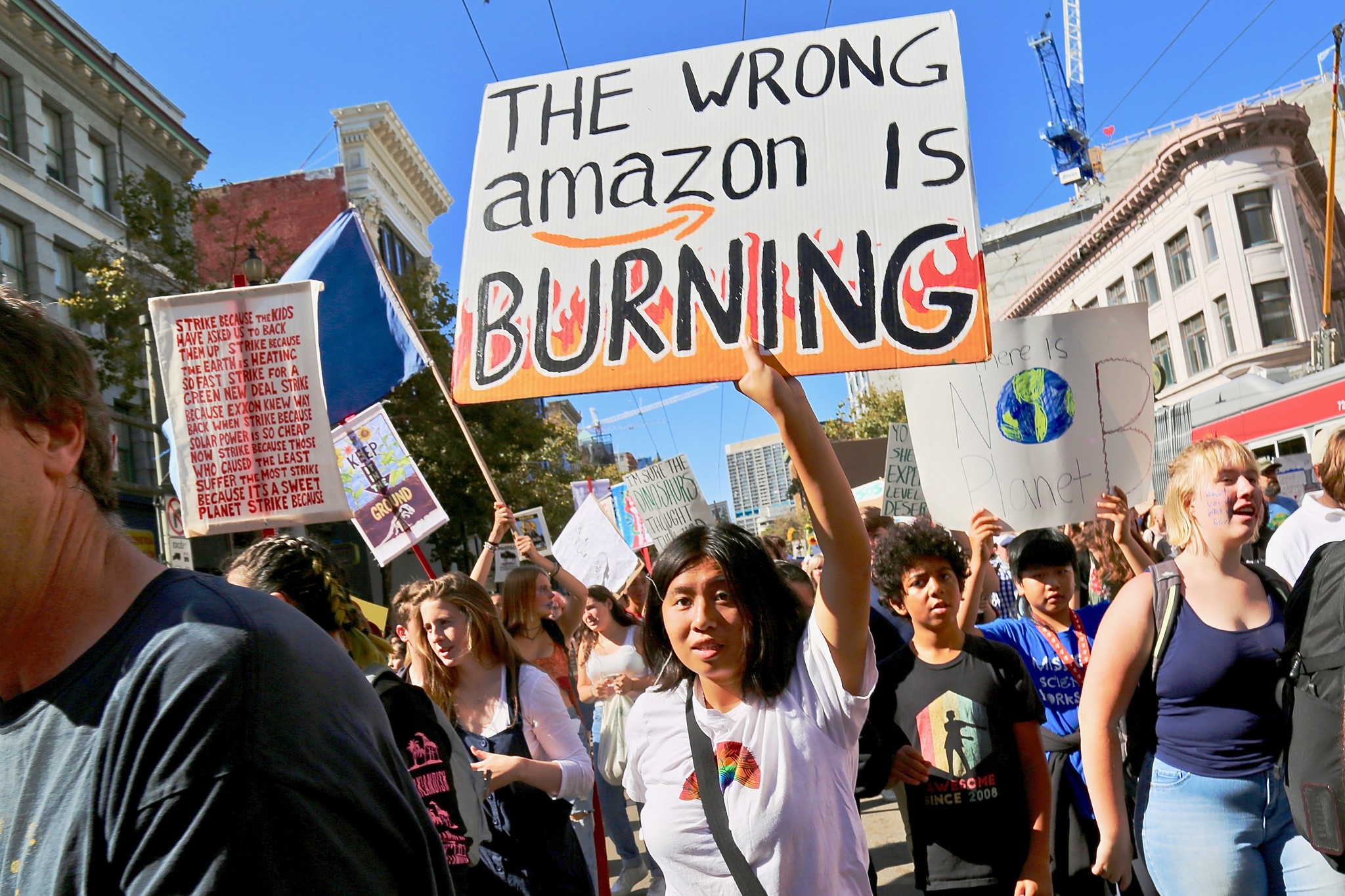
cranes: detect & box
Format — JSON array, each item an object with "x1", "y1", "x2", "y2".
[{"x1": 1024, "y1": 0, "x2": 1104, "y2": 203}]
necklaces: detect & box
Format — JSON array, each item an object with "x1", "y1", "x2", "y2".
[
  {"x1": 526, "y1": 621, "x2": 543, "y2": 631},
  {"x1": 461, "y1": 661, "x2": 498, "y2": 705},
  {"x1": 520, "y1": 626, "x2": 542, "y2": 640}
]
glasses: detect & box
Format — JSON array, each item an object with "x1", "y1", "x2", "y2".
[{"x1": 1262, "y1": 469, "x2": 1276, "y2": 477}]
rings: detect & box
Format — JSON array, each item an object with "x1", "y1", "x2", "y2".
[
  {"x1": 619, "y1": 691, "x2": 621, "y2": 695},
  {"x1": 502, "y1": 513, "x2": 508, "y2": 518}
]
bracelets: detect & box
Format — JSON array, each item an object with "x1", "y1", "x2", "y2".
[
  {"x1": 551, "y1": 564, "x2": 560, "y2": 578},
  {"x1": 591, "y1": 686, "x2": 596, "y2": 697},
  {"x1": 483, "y1": 542, "x2": 497, "y2": 552},
  {"x1": 548, "y1": 562, "x2": 557, "y2": 575},
  {"x1": 487, "y1": 540, "x2": 499, "y2": 546}
]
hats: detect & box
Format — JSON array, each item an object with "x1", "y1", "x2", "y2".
[
  {"x1": 1256, "y1": 459, "x2": 1282, "y2": 474},
  {"x1": 995, "y1": 533, "x2": 1016, "y2": 546},
  {"x1": 1310, "y1": 424, "x2": 1345, "y2": 465}
]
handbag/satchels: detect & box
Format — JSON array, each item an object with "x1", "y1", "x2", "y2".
[{"x1": 596, "y1": 673, "x2": 635, "y2": 787}]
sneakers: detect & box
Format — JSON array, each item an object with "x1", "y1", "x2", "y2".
[
  {"x1": 610, "y1": 858, "x2": 648, "y2": 896},
  {"x1": 646, "y1": 876, "x2": 666, "y2": 896}
]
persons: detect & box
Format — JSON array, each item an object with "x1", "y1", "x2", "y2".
[
  {"x1": 215, "y1": 330, "x2": 1345, "y2": 896},
  {"x1": 0, "y1": 298, "x2": 450, "y2": 896}
]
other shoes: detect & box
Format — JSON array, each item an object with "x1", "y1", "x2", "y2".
[{"x1": 881, "y1": 788, "x2": 897, "y2": 802}]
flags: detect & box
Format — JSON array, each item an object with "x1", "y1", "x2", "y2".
[{"x1": 275, "y1": 209, "x2": 429, "y2": 426}]
